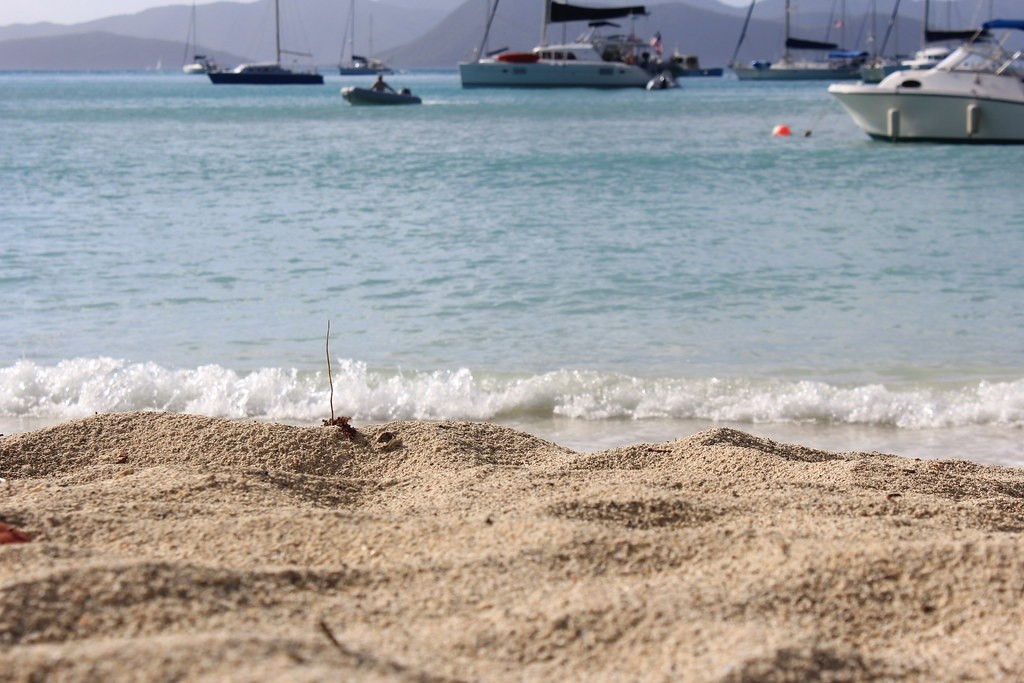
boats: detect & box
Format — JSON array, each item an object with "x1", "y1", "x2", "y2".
[
  {"x1": 340, "y1": 85, "x2": 422, "y2": 106},
  {"x1": 827, "y1": 17, "x2": 1024, "y2": 147}
]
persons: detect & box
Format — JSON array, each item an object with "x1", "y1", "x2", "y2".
[{"x1": 370, "y1": 76, "x2": 394, "y2": 93}]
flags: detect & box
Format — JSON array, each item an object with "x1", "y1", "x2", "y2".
[
  {"x1": 649, "y1": 32, "x2": 663, "y2": 55},
  {"x1": 834, "y1": 20, "x2": 843, "y2": 28}
]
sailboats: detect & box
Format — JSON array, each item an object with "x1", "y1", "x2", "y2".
[
  {"x1": 336, "y1": 0, "x2": 399, "y2": 77},
  {"x1": 181, "y1": 0, "x2": 219, "y2": 76},
  {"x1": 457, "y1": 0, "x2": 725, "y2": 89},
  {"x1": 204, "y1": 0, "x2": 326, "y2": 86},
  {"x1": 729, "y1": 1, "x2": 1024, "y2": 88}
]
